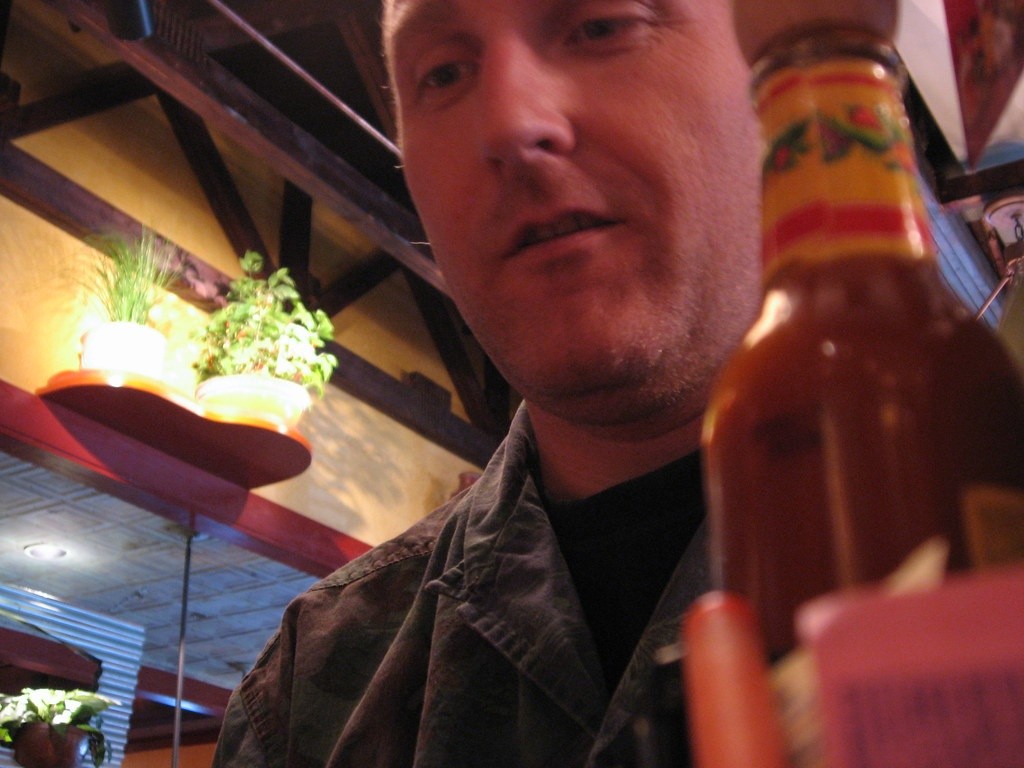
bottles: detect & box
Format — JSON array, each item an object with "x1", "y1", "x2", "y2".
[{"x1": 700, "y1": 1, "x2": 1024, "y2": 768}]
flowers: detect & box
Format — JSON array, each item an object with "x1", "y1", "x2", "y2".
[{"x1": 0, "y1": 687, "x2": 122, "y2": 768}]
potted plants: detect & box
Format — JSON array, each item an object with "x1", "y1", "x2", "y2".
[
  {"x1": 72, "y1": 222, "x2": 188, "y2": 381},
  {"x1": 190, "y1": 249, "x2": 340, "y2": 430}
]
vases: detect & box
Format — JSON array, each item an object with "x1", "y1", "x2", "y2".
[{"x1": 11, "y1": 722, "x2": 89, "y2": 768}]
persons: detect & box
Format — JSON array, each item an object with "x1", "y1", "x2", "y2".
[{"x1": 212, "y1": 0, "x2": 1024, "y2": 768}]
